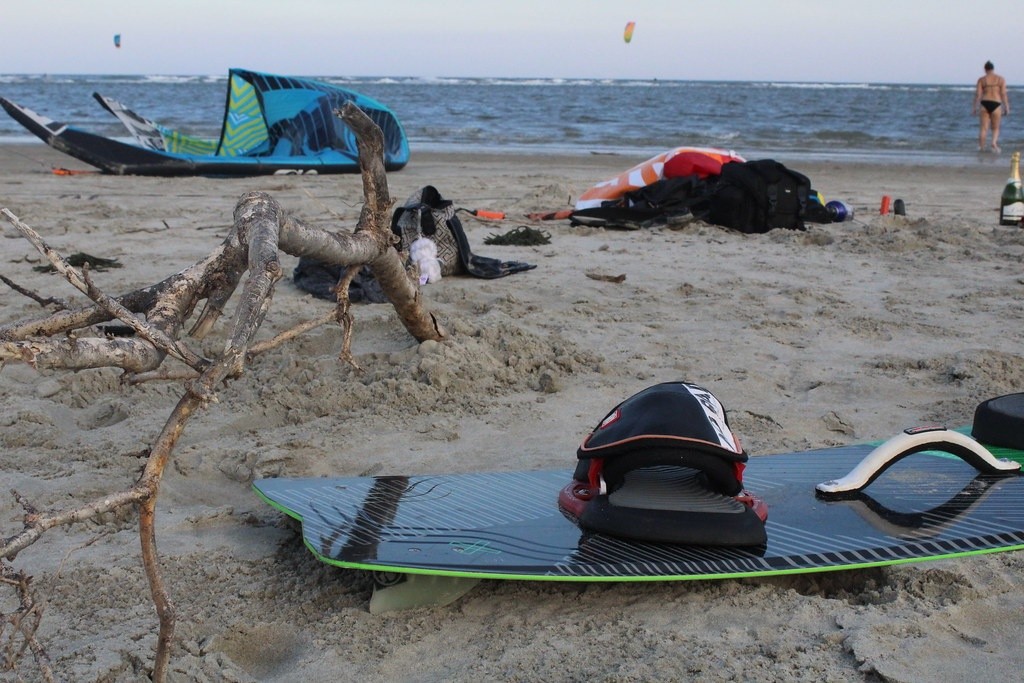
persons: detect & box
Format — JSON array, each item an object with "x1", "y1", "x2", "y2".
[{"x1": 973, "y1": 62, "x2": 1009, "y2": 148}]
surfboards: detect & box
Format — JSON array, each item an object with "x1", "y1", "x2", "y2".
[{"x1": 248, "y1": 423, "x2": 1023, "y2": 583}]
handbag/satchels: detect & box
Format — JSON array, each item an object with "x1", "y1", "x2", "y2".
[
  {"x1": 391, "y1": 185, "x2": 462, "y2": 275},
  {"x1": 709, "y1": 159, "x2": 812, "y2": 235}
]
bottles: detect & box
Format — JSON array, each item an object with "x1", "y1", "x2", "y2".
[
  {"x1": 826, "y1": 201, "x2": 854, "y2": 222},
  {"x1": 1000, "y1": 152, "x2": 1024, "y2": 226}
]
